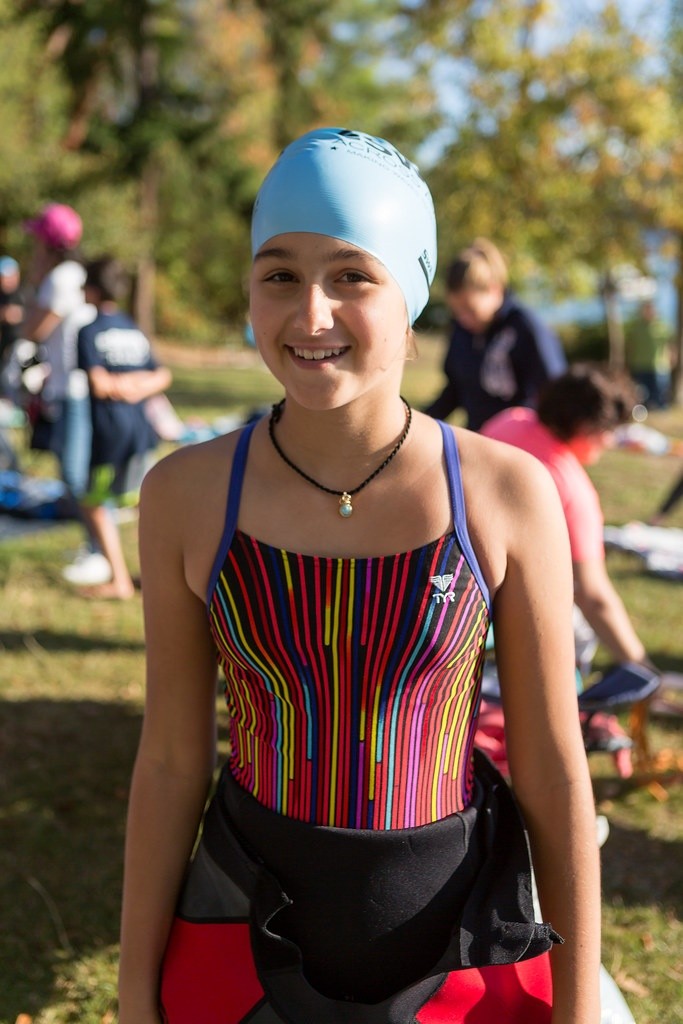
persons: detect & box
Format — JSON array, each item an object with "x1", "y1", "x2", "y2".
[
  {"x1": 114, "y1": 127, "x2": 635, "y2": 1023},
  {"x1": 424, "y1": 237, "x2": 649, "y2": 667},
  {"x1": 1, "y1": 202, "x2": 193, "y2": 602}
]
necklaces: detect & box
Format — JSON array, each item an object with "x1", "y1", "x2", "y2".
[{"x1": 269, "y1": 395, "x2": 412, "y2": 518}]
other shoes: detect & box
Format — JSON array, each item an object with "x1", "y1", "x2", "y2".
[{"x1": 61, "y1": 552, "x2": 112, "y2": 585}]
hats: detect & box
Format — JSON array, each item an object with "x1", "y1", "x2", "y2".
[
  {"x1": 23, "y1": 202, "x2": 82, "y2": 250},
  {"x1": 0, "y1": 255, "x2": 20, "y2": 276},
  {"x1": 250, "y1": 128, "x2": 438, "y2": 332}
]
